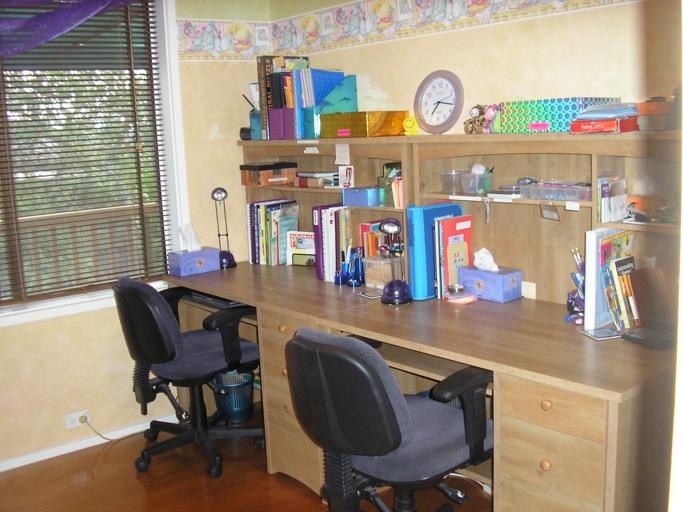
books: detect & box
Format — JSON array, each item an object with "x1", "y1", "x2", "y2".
[
  {"x1": 244, "y1": 197, "x2": 343, "y2": 283},
  {"x1": 406, "y1": 198, "x2": 477, "y2": 300},
  {"x1": 584, "y1": 175, "x2": 650, "y2": 333},
  {"x1": 256, "y1": 55, "x2": 343, "y2": 140},
  {"x1": 360, "y1": 218, "x2": 397, "y2": 259},
  {"x1": 293, "y1": 171, "x2": 339, "y2": 189}
]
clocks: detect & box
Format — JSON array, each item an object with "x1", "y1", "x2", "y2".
[{"x1": 412, "y1": 69, "x2": 464, "y2": 135}]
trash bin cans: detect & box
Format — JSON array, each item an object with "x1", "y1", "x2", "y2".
[{"x1": 214, "y1": 370, "x2": 255, "y2": 424}]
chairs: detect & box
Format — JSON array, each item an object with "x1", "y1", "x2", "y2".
[{"x1": 112, "y1": 279, "x2": 264, "y2": 479}]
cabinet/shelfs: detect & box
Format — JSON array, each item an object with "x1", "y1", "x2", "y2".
[{"x1": 164, "y1": 131, "x2": 678, "y2": 510}]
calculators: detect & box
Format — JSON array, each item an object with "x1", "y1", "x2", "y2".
[{"x1": 622, "y1": 320, "x2": 677, "y2": 349}]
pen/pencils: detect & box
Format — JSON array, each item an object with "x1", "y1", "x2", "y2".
[
  {"x1": 242, "y1": 94, "x2": 255, "y2": 109},
  {"x1": 341, "y1": 238, "x2": 364, "y2": 283},
  {"x1": 565, "y1": 248, "x2": 584, "y2": 325}
]
